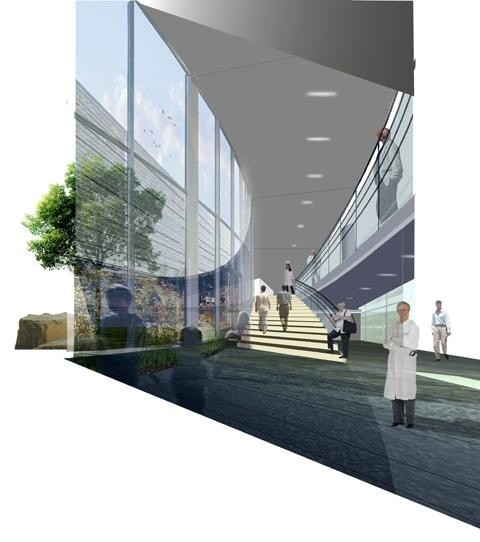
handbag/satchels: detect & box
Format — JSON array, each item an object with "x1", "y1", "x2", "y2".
[{"x1": 343, "y1": 320, "x2": 356, "y2": 332}]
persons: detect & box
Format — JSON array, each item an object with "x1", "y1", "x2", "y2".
[
  {"x1": 173, "y1": 322, "x2": 210, "y2": 413},
  {"x1": 383, "y1": 301, "x2": 420, "y2": 427},
  {"x1": 373, "y1": 127, "x2": 403, "y2": 224},
  {"x1": 277, "y1": 262, "x2": 297, "y2": 296},
  {"x1": 255, "y1": 284, "x2": 272, "y2": 334},
  {"x1": 275, "y1": 284, "x2": 293, "y2": 333},
  {"x1": 306, "y1": 249, "x2": 322, "y2": 288},
  {"x1": 328, "y1": 301, "x2": 358, "y2": 358},
  {"x1": 429, "y1": 300, "x2": 453, "y2": 361},
  {"x1": 93, "y1": 287, "x2": 146, "y2": 385}
]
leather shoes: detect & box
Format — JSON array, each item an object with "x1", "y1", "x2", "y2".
[
  {"x1": 390, "y1": 422, "x2": 403, "y2": 427},
  {"x1": 407, "y1": 424, "x2": 413, "y2": 429},
  {"x1": 339, "y1": 356, "x2": 347, "y2": 358},
  {"x1": 435, "y1": 355, "x2": 448, "y2": 361},
  {"x1": 327, "y1": 350, "x2": 334, "y2": 353}
]
